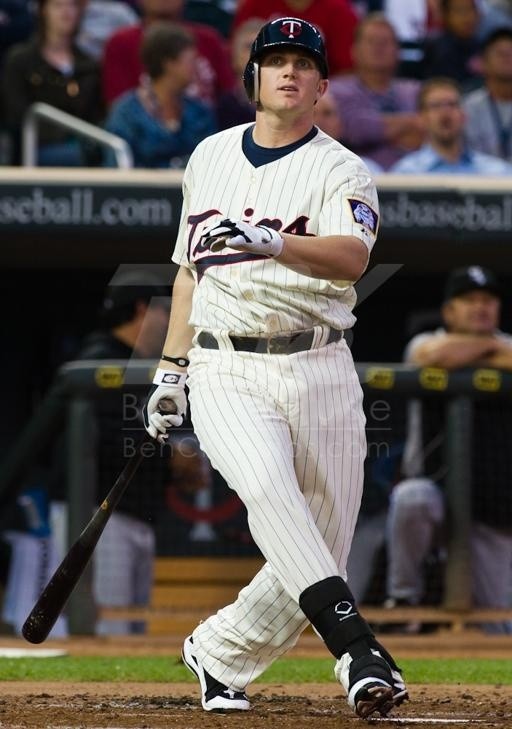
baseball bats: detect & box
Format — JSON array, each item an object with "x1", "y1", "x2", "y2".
[{"x1": 22, "y1": 397, "x2": 177, "y2": 644}]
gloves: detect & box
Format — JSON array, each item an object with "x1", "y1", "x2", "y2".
[
  {"x1": 142, "y1": 367, "x2": 188, "y2": 443},
  {"x1": 199, "y1": 219, "x2": 284, "y2": 257}
]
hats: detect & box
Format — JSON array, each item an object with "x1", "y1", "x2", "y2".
[{"x1": 443, "y1": 266, "x2": 504, "y2": 303}]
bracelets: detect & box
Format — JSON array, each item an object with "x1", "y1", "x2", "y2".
[{"x1": 158, "y1": 353, "x2": 189, "y2": 369}]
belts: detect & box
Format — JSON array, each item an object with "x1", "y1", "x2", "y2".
[{"x1": 197, "y1": 326, "x2": 342, "y2": 353}]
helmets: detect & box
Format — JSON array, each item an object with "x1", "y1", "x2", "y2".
[{"x1": 244, "y1": 17, "x2": 328, "y2": 105}]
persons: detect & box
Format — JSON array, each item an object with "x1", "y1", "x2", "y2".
[
  {"x1": 1, "y1": 2, "x2": 510, "y2": 176},
  {"x1": 142, "y1": 13, "x2": 410, "y2": 720},
  {"x1": 384, "y1": 265, "x2": 512, "y2": 634},
  {"x1": 2, "y1": 247, "x2": 246, "y2": 643}
]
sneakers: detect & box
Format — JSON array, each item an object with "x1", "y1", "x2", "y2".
[
  {"x1": 180, "y1": 635, "x2": 251, "y2": 714},
  {"x1": 336, "y1": 640, "x2": 408, "y2": 717}
]
